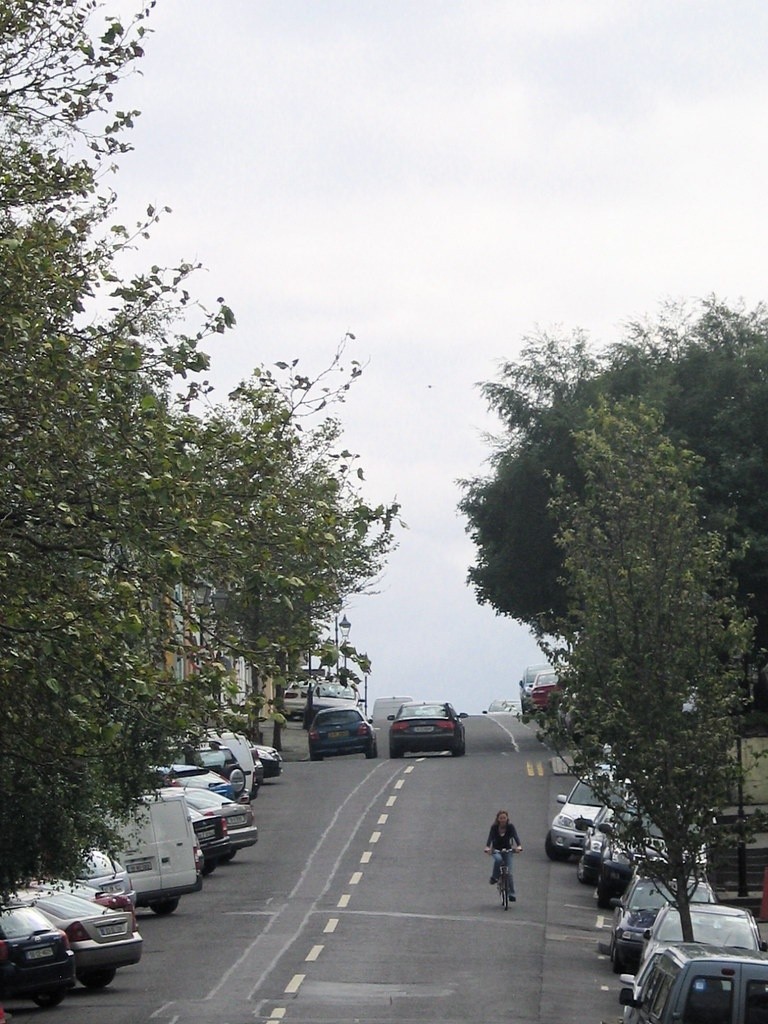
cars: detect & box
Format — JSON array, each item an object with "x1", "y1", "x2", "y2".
[
  {"x1": 308, "y1": 707, "x2": 378, "y2": 761},
  {"x1": 283, "y1": 681, "x2": 316, "y2": 720},
  {"x1": 640, "y1": 900, "x2": 768, "y2": 969},
  {"x1": 145, "y1": 786, "x2": 258, "y2": 864},
  {"x1": 29, "y1": 879, "x2": 140, "y2": 932},
  {"x1": 302, "y1": 680, "x2": 363, "y2": 729},
  {"x1": 482, "y1": 699, "x2": 522, "y2": 715},
  {"x1": 76, "y1": 848, "x2": 137, "y2": 905},
  {"x1": 529, "y1": 672, "x2": 558, "y2": 712},
  {"x1": 254, "y1": 744, "x2": 283, "y2": 778},
  {"x1": 386, "y1": 702, "x2": 469, "y2": 759},
  {"x1": 575, "y1": 805, "x2": 618, "y2": 884},
  {"x1": 188, "y1": 806, "x2": 231, "y2": 875},
  {"x1": 0, "y1": 889, "x2": 144, "y2": 989},
  {"x1": 0, "y1": 902, "x2": 76, "y2": 1010},
  {"x1": 597, "y1": 808, "x2": 707, "y2": 908},
  {"x1": 619, "y1": 942, "x2": 710, "y2": 1015}
]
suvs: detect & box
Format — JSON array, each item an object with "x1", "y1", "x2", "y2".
[
  {"x1": 608, "y1": 874, "x2": 717, "y2": 974},
  {"x1": 519, "y1": 664, "x2": 554, "y2": 714},
  {"x1": 150, "y1": 764, "x2": 235, "y2": 802},
  {"x1": 167, "y1": 741, "x2": 250, "y2": 804},
  {"x1": 544, "y1": 764, "x2": 631, "y2": 861}
]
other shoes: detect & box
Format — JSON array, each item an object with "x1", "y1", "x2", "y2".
[
  {"x1": 489, "y1": 877, "x2": 496, "y2": 884},
  {"x1": 509, "y1": 896, "x2": 516, "y2": 902}
]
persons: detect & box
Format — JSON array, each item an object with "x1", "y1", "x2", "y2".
[{"x1": 485, "y1": 810, "x2": 522, "y2": 902}]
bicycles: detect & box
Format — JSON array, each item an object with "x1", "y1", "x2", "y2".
[{"x1": 484, "y1": 849, "x2": 524, "y2": 911}]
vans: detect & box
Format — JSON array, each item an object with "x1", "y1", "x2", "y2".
[
  {"x1": 618, "y1": 945, "x2": 768, "y2": 1024},
  {"x1": 205, "y1": 729, "x2": 258, "y2": 801},
  {"x1": 371, "y1": 697, "x2": 413, "y2": 729},
  {"x1": 93, "y1": 795, "x2": 203, "y2": 916}
]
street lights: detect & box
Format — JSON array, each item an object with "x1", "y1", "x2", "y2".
[
  {"x1": 339, "y1": 614, "x2": 351, "y2": 668},
  {"x1": 208, "y1": 591, "x2": 230, "y2": 708},
  {"x1": 192, "y1": 579, "x2": 212, "y2": 644}
]
